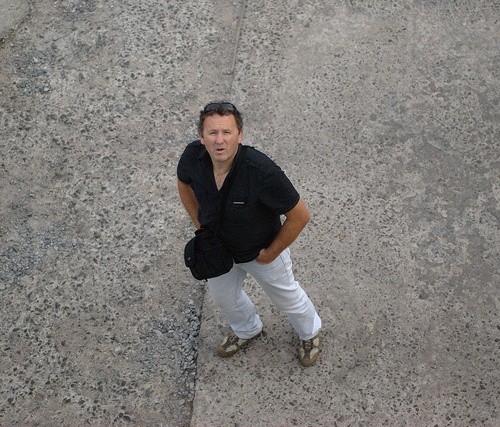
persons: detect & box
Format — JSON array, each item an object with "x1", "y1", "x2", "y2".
[{"x1": 177, "y1": 99, "x2": 324, "y2": 368}]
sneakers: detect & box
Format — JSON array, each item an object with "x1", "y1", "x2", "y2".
[
  {"x1": 217, "y1": 332, "x2": 251, "y2": 358},
  {"x1": 299, "y1": 331, "x2": 319, "y2": 367}
]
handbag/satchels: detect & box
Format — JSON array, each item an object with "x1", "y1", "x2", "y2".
[{"x1": 183, "y1": 227, "x2": 234, "y2": 281}]
